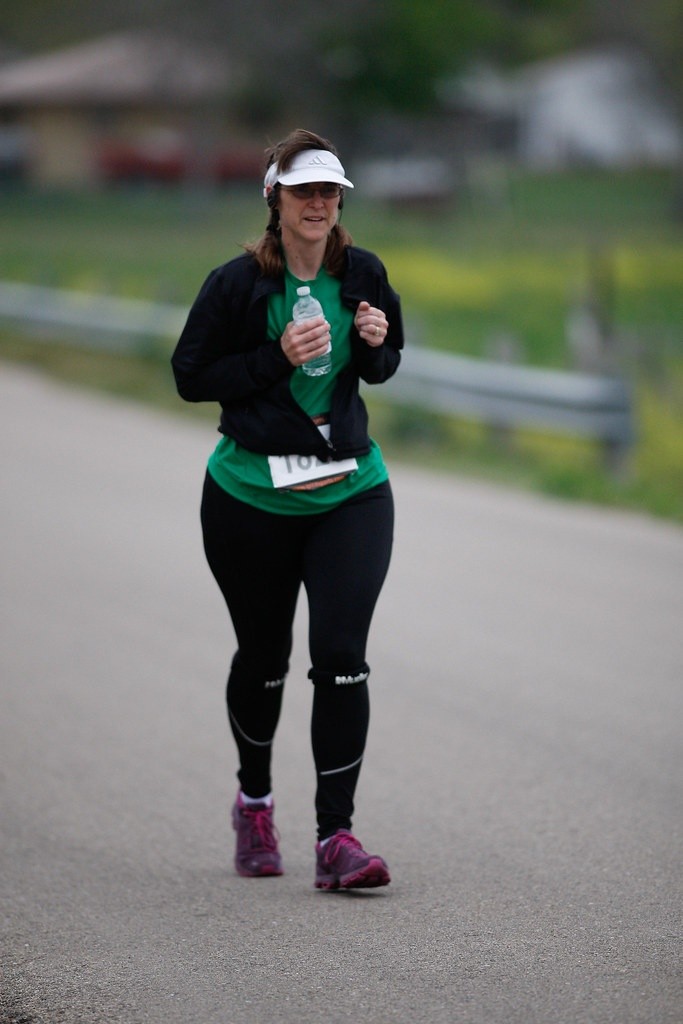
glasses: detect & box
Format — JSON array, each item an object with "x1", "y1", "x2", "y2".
[{"x1": 274, "y1": 182, "x2": 342, "y2": 199}]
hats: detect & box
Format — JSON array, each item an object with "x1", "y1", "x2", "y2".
[{"x1": 263, "y1": 148, "x2": 354, "y2": 198}]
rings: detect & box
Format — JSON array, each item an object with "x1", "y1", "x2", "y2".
[{"x1": 375, "y1": 326, "x2": 379, "y2": 336}]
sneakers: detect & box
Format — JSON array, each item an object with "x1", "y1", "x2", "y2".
[
  {"x1": 231, "y1": 788, "x2": 285, "y2": 875},
  {"x1": 314, "y1": 828, "x2": 391, "y2": 889}
]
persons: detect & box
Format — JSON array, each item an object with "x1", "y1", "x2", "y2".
[{"x1": 173, "y1": 130, "x2": 407, "y2": 891}]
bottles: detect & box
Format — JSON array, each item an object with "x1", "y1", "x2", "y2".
[{"x1": 293, "y1": 285, "x2": 332, "y2": 376}]
